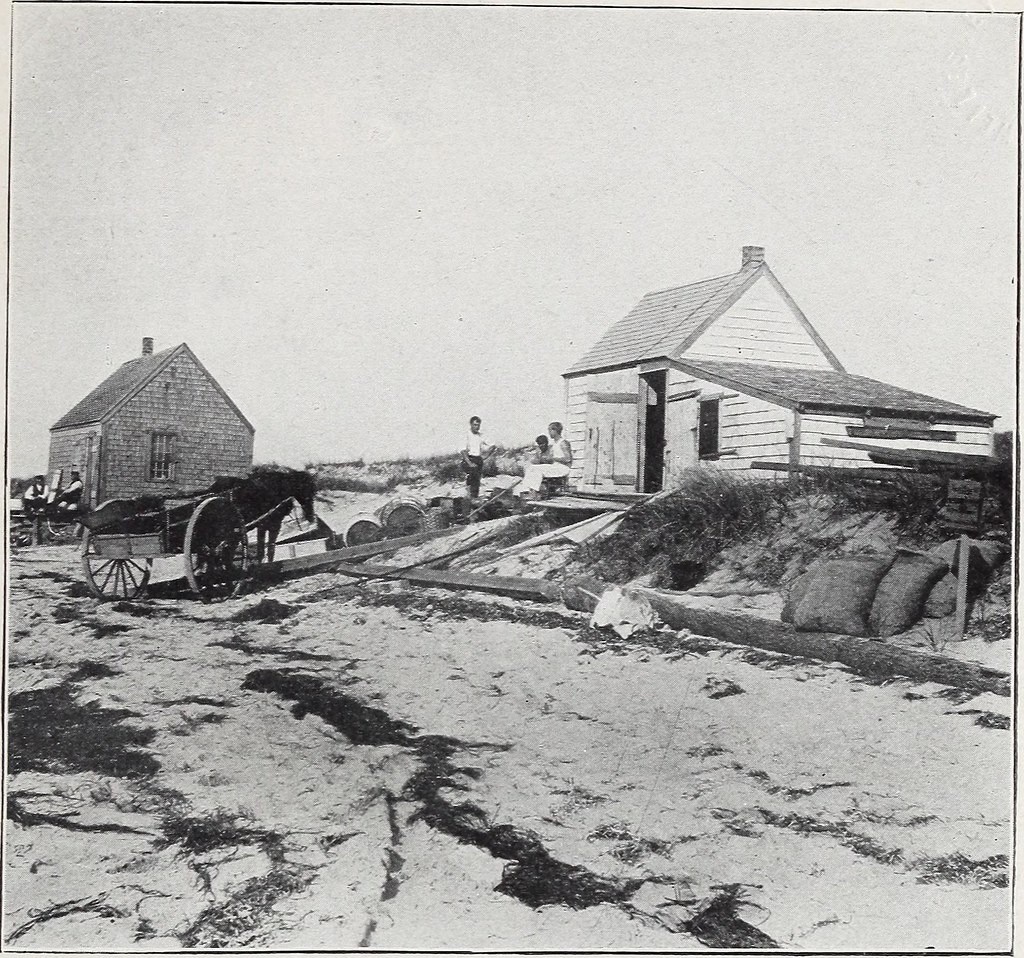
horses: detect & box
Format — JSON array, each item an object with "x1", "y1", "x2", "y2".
[{"x1": 208, "y1": 468, "x2": 318, "y2": 564}]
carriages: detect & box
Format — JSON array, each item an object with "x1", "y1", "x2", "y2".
[{"x1": 73, "y1": 460, "x2": 318, "y2": 605}]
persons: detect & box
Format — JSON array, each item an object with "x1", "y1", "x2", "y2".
[
  {"x1": 522, "y1": 422, "x2": 573, "y2": 501},
  {"x1": 458, "y1": 416, "x2": 497, "y2": 504},
  {"x1": 23, "y1": 470, "x2": 82, "y2": 520}
]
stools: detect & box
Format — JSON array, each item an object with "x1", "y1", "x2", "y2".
[{"x1": 546, "y1": 477, "x2": 566, "y2": 500}]
